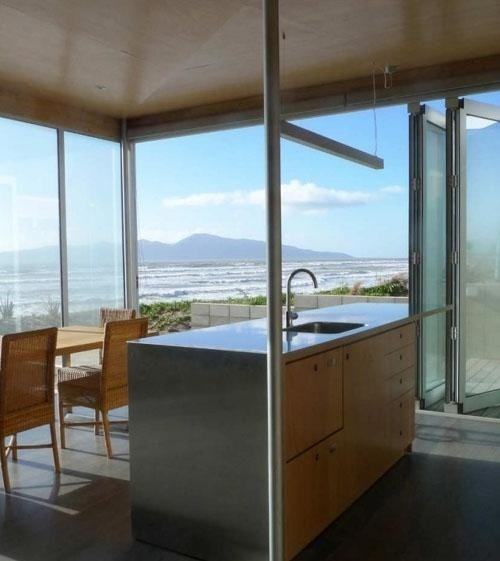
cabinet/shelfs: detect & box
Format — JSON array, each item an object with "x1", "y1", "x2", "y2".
[
  {"x1": 342, "y1": 331, "x2": 385, "y2": 514},
  {"x1": 385, "y1": 321, "x2": 418, "y2": 476},
  {"x1": 284, "y1": 346, "x2": 344, "y2": 561}
]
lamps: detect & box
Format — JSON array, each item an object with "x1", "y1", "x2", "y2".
[{"x1": 279, "y1": 31, "x2": 384, "y2": 172}]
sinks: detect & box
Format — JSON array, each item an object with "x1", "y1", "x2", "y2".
[{"x1": 287, "y1": 322, "x2": 365, "y2": 334}]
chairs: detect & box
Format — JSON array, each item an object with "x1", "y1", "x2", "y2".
[
  {"x1": 0, "y1": 326, "x2": 62, "y2": 494},
  {"x1": 57, "y1": 306, "x2": 136, "y2": 435},
  {"x1": 57, "y1": 314, "x2": 150, "y2": 461}
]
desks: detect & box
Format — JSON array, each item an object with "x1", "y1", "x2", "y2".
[{"x1": 0, "y1": 324, "x2": 160, "y2": 461}]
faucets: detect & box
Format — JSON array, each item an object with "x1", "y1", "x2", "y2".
[{"x1": 286, "y1": 268, "x2": 318, "y2": 329}]
